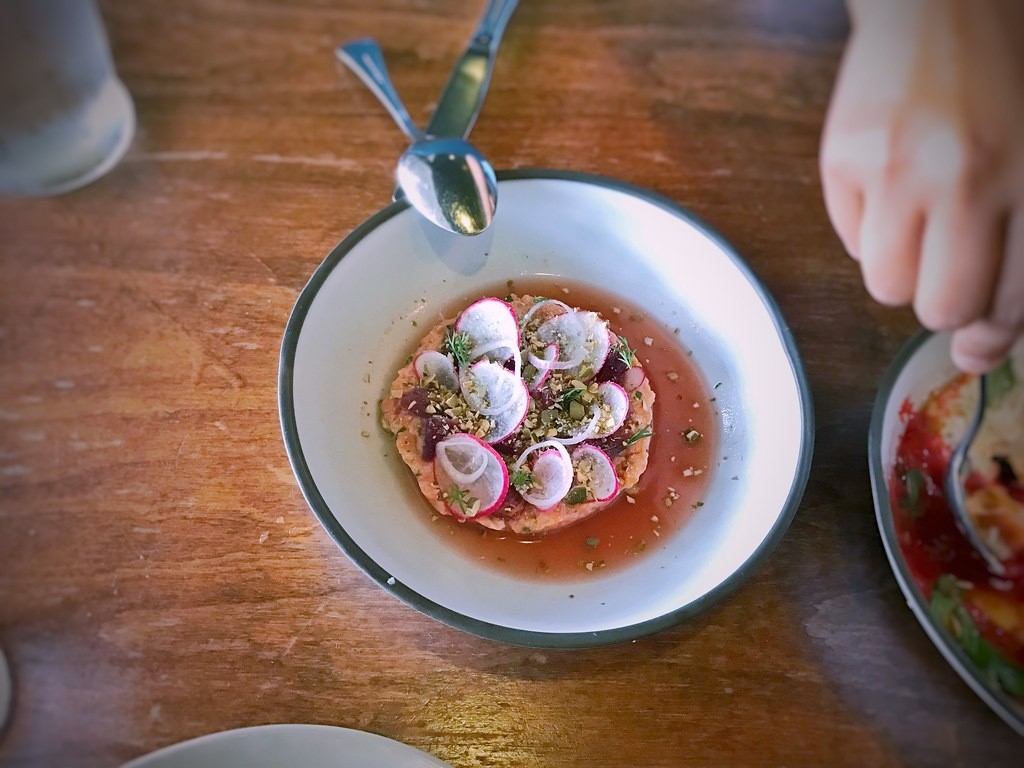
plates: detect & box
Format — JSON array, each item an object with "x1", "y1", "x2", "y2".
[
  {"x1": 109, "y1": 724, "x2": 455, "y2": 768},
  {"x1": 866, "y1": 315, "x2": 1024, "y2": 743}
]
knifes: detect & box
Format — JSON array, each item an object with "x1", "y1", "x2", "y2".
[{"x1": 392, "y1": 0, "x2": 524, "y2": 204}]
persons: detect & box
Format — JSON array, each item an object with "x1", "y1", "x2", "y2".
[{"x1": 818, "y1": 0, "x2": 1024, "y2": 373}]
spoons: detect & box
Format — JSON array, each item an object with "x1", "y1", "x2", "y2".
[
  {"x1": 333, "y1": 36, "x2": 498, "y2": 237},
  {"x1": 943, "y1": 371, "x2": 1009, "y2": 577}
]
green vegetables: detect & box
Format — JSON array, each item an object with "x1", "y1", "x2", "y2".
[{"x1": 439, "y1": 295, "x2": 653, "y2": 517}]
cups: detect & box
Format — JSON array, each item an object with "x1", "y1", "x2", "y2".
[{"x1": 0, "y1": 0, "x2": 138, "y2": 199}]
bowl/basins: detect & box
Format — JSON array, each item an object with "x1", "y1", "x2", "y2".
[{"x1": 278, "y1": 172, "x2": 815, "y2": 649}]
